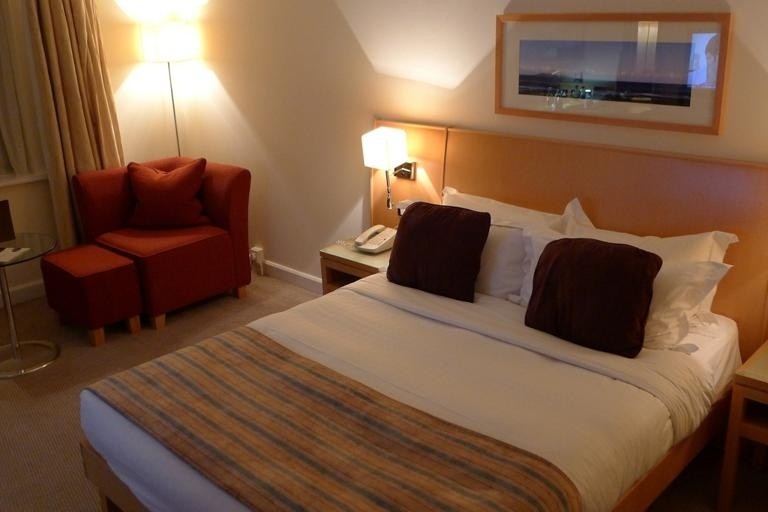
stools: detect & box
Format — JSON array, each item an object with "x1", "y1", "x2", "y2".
[{"x1": 40, "y1": 241, "x2": 144, "y2": 347}]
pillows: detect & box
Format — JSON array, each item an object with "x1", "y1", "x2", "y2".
[
  {"x1": 440, "y1": 185, "x2": 562, "y2": 230},
  {"x1": 125, "y1": 157, "x2": 211, "y2": 228},
  {"x1": 506, "y1": 222, "x2": 735, "y2": 355},
  {"x1": 386, "y1": 200, "x2": 492, "y2": 304},
  {"x1": 523, "y1": 237, "x2": 664, "y2": 360},
  {"x1": 398, "y1": 199, "x2": 527, "y2": 299},
  {"x1": 546, "y1": 197, "x2": 740, "y2": 337}
]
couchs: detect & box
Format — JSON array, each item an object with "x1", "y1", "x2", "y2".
[{"x1": 72, "y1": 156, "x2": 253, "y2": 331}]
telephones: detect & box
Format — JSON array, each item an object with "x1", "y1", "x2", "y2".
[{"x1": 335, "y1": 224, "x2": 397, "y2": 254}]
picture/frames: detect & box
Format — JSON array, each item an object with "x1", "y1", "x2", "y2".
[{"x1": 494, "y1": 11, "x2": 732, "y2": 137}]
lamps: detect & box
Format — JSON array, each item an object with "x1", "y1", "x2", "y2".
[
  {"x1": 133, "y1": 18, "x2": 206, "y2": 157},
  {"x1": 360, "y1": 125, "x2": 413, "y2": 217}
]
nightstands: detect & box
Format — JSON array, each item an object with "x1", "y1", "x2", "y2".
[
  {"x1": 720, "y1": 338, "x2": 768, "y2": 512},
  {"x1": 319, "y1": 234, "x2": 393, "y2": 296}
]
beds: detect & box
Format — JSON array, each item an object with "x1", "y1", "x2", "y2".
[{"x1": 78, "y1": 116, "x2": 767, "y2": 512}]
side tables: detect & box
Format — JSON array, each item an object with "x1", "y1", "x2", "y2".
[{"x1": 0, "y1": 231, "x2": 61, "y2": 379}]
